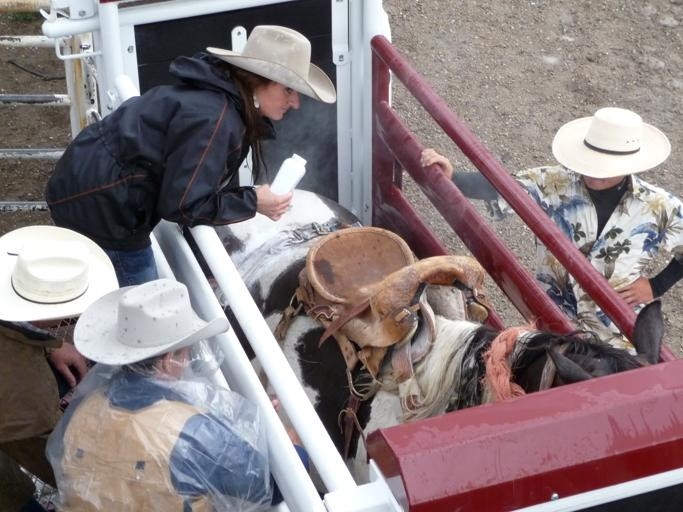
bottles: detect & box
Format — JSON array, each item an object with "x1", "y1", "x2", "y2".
[{"x1": 269, "y1": 152, "x2": 308, "y2": 197}]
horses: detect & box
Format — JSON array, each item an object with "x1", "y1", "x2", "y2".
[{"x1": 180, "y1": 179, "x2": 683, "y2": 512}]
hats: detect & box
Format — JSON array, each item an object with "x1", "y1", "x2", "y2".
[
  {"x1": 0, "y1": 224, "x2": 120, "y2": 323},
  {"x1": 73, "y1": 278, "x2": 228, "y2": 367},
  {"x1": 552, "y1": 107, "x2": 671, "y2": 180},
  {"x1": 206, "y1": 26, "x2": 337, "y2": 105}
]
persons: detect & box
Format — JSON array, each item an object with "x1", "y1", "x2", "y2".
[
  {"x1": 46, "y1": 277, "x2": 311, "y2": 512},
  {"x1": 47, "y1": 25, "x2": 337, "y2": 288},
  {"x1": 418, "y1": 107, "x2": 683, "y2": 358},
  {"x1": 0, "y1": 224, "x2": 121, "y2": 512}
]
